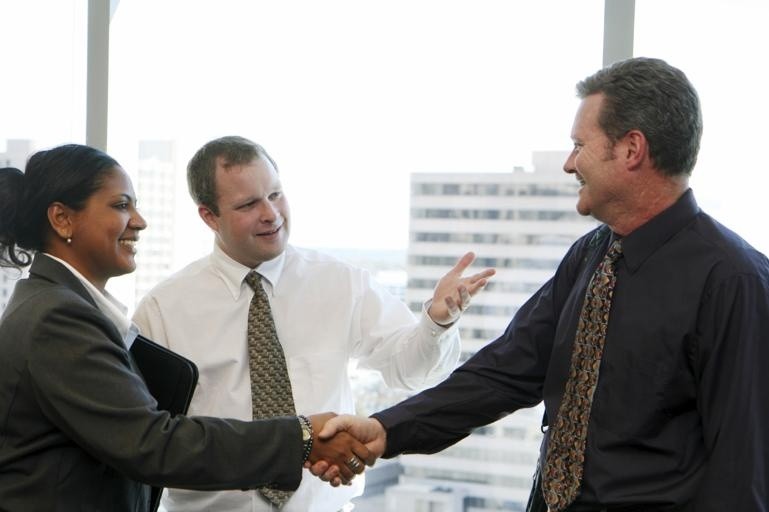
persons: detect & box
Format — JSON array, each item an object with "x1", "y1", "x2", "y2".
[
  {"x1": 304, "y1": 58, "x2": 769, "y2": 512},
  {"x1": 132, "y1": 136, "x2": 495, "y2": 512},
  {"x1": 0, "y1": 144, "x2": 374, "y2": 511}
]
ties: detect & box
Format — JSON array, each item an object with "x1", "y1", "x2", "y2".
[
  {"x1": 246, "y1": 270, "x2": 302, "y2": 511},
  {"x1": 540, "y1": 241, "x2": 619, "y2": 511}
]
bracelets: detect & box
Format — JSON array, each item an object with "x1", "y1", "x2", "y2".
[{"x1": 298, "y1": 416, "x2": 314, "y2": 461}]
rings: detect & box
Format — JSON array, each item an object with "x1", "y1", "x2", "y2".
[{"x1": 347, "y1": 455, "x2": 361, "y2": 471}]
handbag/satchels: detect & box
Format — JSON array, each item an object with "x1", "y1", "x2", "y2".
[{"x1": 126, "y1": 333, "x2": 199, "y2": 511}]
